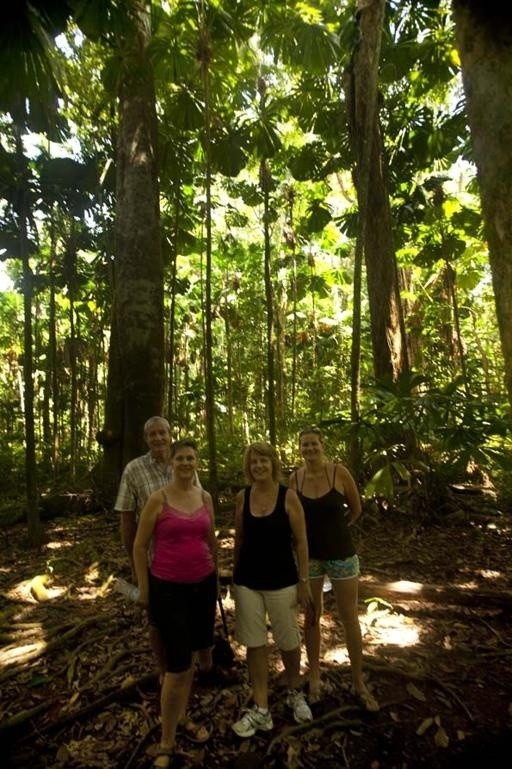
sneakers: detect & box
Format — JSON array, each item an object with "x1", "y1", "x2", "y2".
[
  {"x1": 308, "y1": 677, "x2": 321, "y2": 703},
  {"x1": 231, "y1": 703, "x2": 273, "y2": 737},
  {"x1": 198, "y1": 668, "x2": 244, "y2": 687},
  {"x1": 287, "y1": 692, "x2": 313, "y2": 724},
  {"x1": 350, "y1": 686, "x2": 379, "y2": 712}
]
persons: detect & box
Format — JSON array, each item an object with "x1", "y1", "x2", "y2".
[
  {"x1": 131, "y1": 439, "x2": 221, "y2": 769},
  {"x1": 229, "y1": 442, "x2": 314, "y2": 739},
  {"x1": 114, "y1": 417, "x2": 228, "y2": 688},
  {"x1": 288, "y1": 429, "x2": 381, "y2": 714}
]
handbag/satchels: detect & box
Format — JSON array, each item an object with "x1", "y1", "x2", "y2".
[{"x1": 212, "y1": 596, "x2": 234, "y2": 666}]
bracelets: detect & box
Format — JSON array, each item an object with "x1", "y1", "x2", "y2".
[{"x1": 299, "y1": 575, "x2": 310, "y2": 583}]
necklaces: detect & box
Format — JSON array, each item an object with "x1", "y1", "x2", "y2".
[{"x1": 260, "y1": 504, "x2": 269, "y2": 515}]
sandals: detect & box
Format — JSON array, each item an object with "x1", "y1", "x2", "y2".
[
  {"x1": 151, "y1": 741, "x2": 176, "y2": 769},
  {"x1": 176, "y1": 716, "x2": 209, "y2": 742}
]
